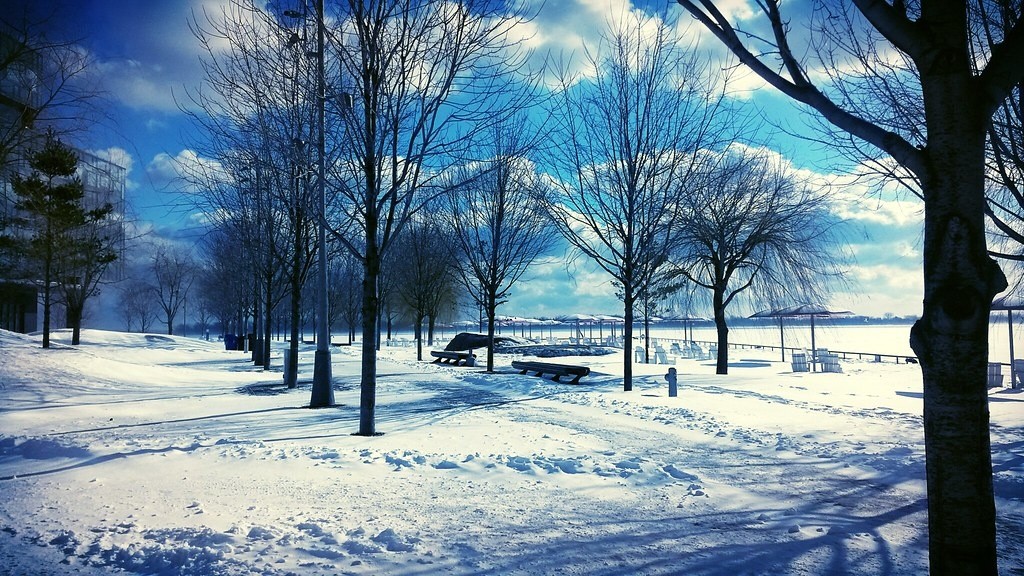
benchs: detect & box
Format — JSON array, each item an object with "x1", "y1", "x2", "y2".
[
  {"x1": 512, "y1": 361, "x2": 590, "y2": 383},
  {"x1": 430, "y1": 351, "x2": 477, "y2": 365}
]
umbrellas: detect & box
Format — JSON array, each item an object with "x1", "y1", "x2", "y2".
[
  {"x1": 749, "y1": 301, "x2": 855, "y2": 372},
  {"x1": 410, "y1": 314, "x2": 714, "y2": 349},
  {"x1": 990, "y1": 295, "x2": 1024, "y2": 388}
]
